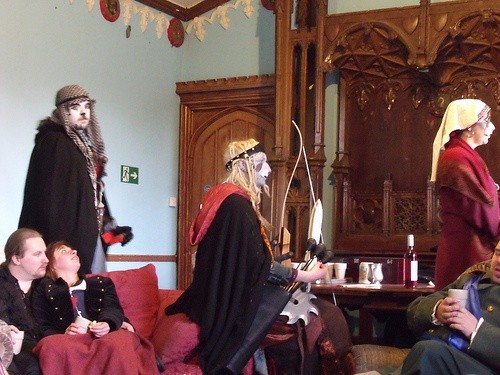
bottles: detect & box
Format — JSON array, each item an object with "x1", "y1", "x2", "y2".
[{"x1": 405, "y1": 235, "x2": 418, "y2": 288}]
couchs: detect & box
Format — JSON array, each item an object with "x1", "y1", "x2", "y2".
[{"x1": 342, "y1": 260, "x2": 491, "y2": 374}]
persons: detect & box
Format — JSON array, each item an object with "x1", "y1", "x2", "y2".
[
  {"x1": 32, "y1": 241, "x2": 158, "y2": 375},
  {"x1": 400, "y1": 241, "x2": 500, "y2": 375},
  {"x1": 17, "y1": 85, "x2": 133, "y2": 273},
  {"x1": 0, "y1": 228, "x2": 74, "y2": 375},
  {"x1": 164, "y1": 139, "x2": 357, "y2": 375},
  {"x1": 430, "y1": 98, "x2": 500, "y2": 290}
]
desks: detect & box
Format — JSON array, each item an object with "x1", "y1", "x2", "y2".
[{"x1": 312, "y1": 281, "x2": 450, "y2": 343}]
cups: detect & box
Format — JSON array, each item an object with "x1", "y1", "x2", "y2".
[
  {"x1": 325, "y1": 263, "x2": 334, "y2": 279},
  {"x1": 359, "y1": 262, "x2": 374, "y2": 283},
  {"x1": 367, "y1": 263, "x2": 383, "y2": 286},
  {"x1": 74, "y1": 315, "x2": 91, "y2": 334},
  {"x1": 448, "y1": 289, "x2": 468, "y2": 308},
  {"x1": 11, "y1": 331, "x2": 24, "y2": 353},
  {"x1": 334, "y1": 263, "x2": 347, "y2": 279}
]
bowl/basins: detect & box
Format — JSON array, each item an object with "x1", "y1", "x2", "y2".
[{"x1": 291, "y1": 263, "x2": 315, "y2": 271}]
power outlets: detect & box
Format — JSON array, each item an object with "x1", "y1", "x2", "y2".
[{"x1": 169, "y1": 196, "x2": 176, "y2": 206}]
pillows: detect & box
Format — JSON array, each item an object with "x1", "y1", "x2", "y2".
[
  {"x1": 156, "y1": 289, "x2": 202, "y2": 366},
  {"x1": 84, "y1": 262, "x2": 161, "y2": 339}
]
